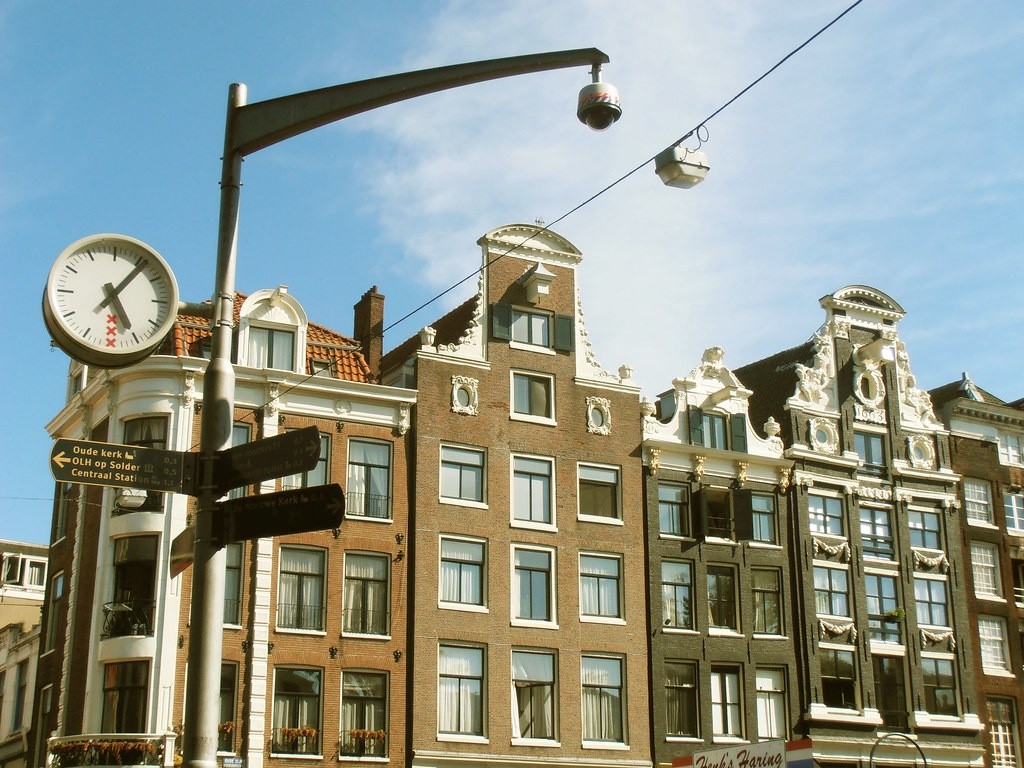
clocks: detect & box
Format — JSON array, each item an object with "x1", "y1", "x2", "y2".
[{"x1": 41, "y1": 233, "x2": 180, "y2": 370}]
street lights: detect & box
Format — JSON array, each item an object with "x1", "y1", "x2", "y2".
[{"x1": 176, "y1": 46, "x2": 623, "y2": 768}]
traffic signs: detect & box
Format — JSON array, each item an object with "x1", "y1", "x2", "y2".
[
  {"x1": 215, "y1": 482, "x2": 345, "y2": 544},
  {"x1": 213, "y1": 424, "x2": 321, "y2": 491},
  {"x1": 50, "y1": 436, "x2": 200, "y2": 495}
]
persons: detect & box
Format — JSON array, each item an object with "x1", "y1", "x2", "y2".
[
  {"x1": 793, "y1": 323, "x2": 833, "y2": 401},
  {"x1": 894, "y1": 340, "x2": 938, "y2": 424}
]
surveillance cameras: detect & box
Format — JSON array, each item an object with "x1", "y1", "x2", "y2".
[{"x1": 577, "y1": 83, "x2": 622, "y2": 131}]
